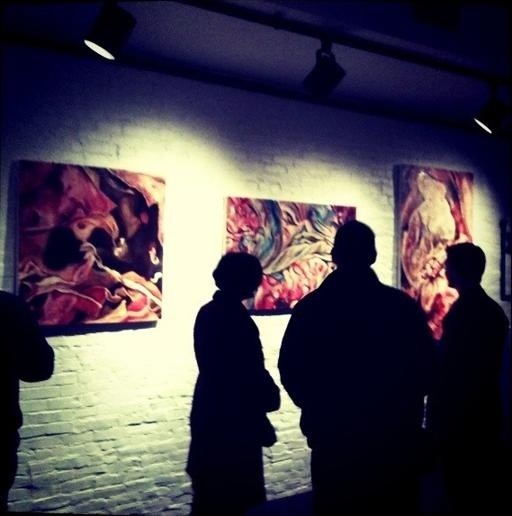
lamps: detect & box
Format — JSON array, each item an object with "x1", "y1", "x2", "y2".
[
  {"x1": 82, "y1": 6, "x2": 137, "y2": 63},
  {"x1": 299, "y1": 46, "x2": 347, "y2": 104},
  {"x1": 473, "y1": 100, "x2": 510, "y2": 137}
]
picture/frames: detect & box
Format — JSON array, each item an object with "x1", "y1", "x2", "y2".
[{"x1": 499, "y1": 215, "x2": 512, "y2": 302}]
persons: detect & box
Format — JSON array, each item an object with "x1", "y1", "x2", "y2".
[
  {"x1": 178, "y1": 250, "x2": 282, "y2": 514},
  {"x1": 276, "y1": 220, "x2": 436, "y2": 513},
  {"x1": 1, "y1": 289, "x2": 55, "y2": 515},
  {"x1": 419, "y1": 239, "x2": 511, "y2": 513}
]
